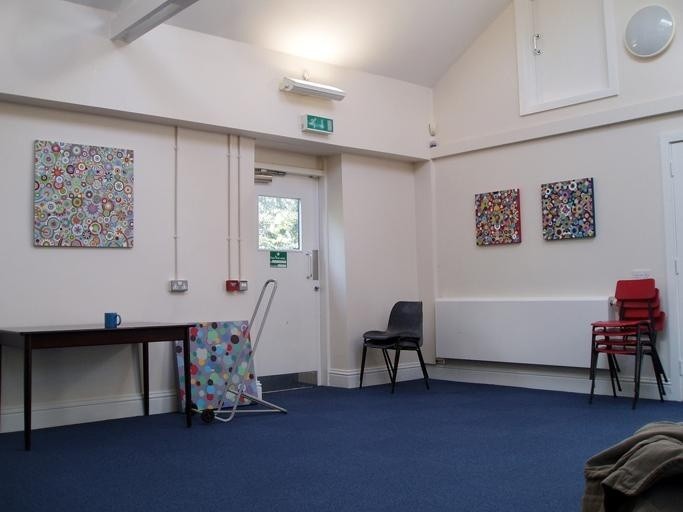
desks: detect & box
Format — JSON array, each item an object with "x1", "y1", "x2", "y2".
[{"x1": 0, "y1": 322, "x2": 197, "y2": 450}]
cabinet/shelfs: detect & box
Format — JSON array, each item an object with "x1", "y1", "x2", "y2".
[{"x1": 515, "y1": 0, "x2": 620, "y2": 116}]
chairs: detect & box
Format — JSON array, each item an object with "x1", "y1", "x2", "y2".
[
  {"x1": 588, "y1": 276, "x2": 668, "y2": 409},
  {"x1": 359, "y1": 301, "x2": 429, "y2": 395}
]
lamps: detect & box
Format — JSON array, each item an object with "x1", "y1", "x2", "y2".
[{"x1": 281, "y1": 78, "x2": 346, "y2": 101}]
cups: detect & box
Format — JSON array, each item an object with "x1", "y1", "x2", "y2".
[{"x1": 104, "y1": 312, "x2": 121, "y2": 329}]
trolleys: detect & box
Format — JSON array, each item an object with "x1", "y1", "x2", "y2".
[{"x1": 185, "y1": 280, "x2": 288, "y2": 423}]
